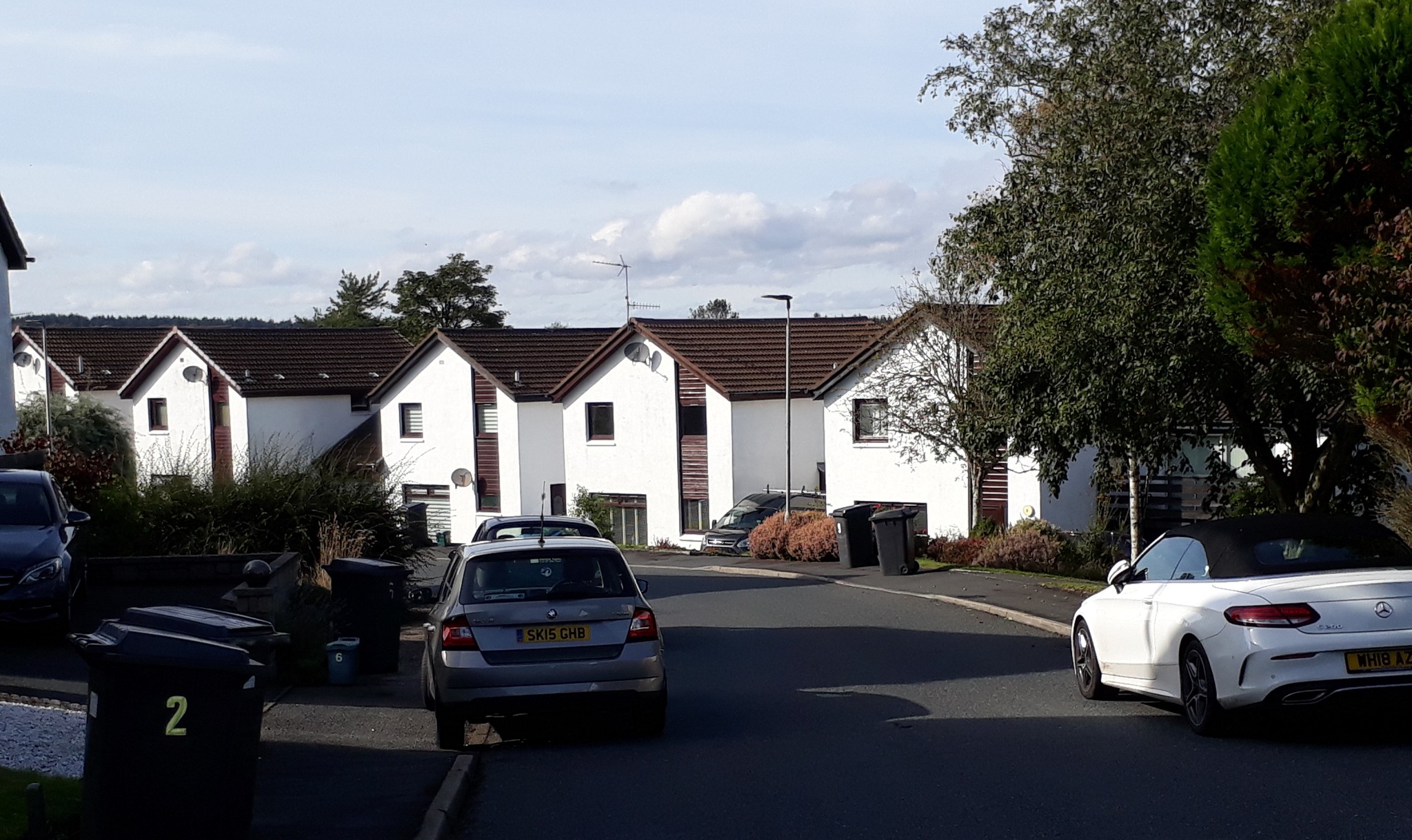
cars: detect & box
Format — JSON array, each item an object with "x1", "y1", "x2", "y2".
[
  {"x1": 421, "y1": 536, "x2": 669, "y2": 750},
  {"x1": 1070, "y1": 513, "x2": 1412, "y2": 738},
  {"x1": 471, "y1": 514, "x2": 603, "y2": 542},
  {"x1": 0, "y1": 468, "x2": 93, "y2": 632}
]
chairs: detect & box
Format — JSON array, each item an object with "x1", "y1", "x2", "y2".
[{"x1": 14, "y1": 486, "x2": 48, "y2": 525}]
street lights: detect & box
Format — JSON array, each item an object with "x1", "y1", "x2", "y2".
[
  {"x1": 24, "y1": 319, "x2": 54, "y2": 464},
  {"x1": 761, "y1": 293, "x2": 794, "y2": 523}
]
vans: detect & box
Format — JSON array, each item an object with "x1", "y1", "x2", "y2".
[{"x1": 700, "y1": 484, "x2": 825, "y2": 554}]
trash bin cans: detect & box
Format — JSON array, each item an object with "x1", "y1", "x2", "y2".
[
  {"x1": 100, "y1": 604, "x2": 290, "y2": 840},
  {"x1": 868, "y1": 508, "x2": 920, "y2": 576},
  {"x1": 397, "y1": 502, "x2": 431, "y2": 547},
  {"x1": 828, "y1": 503, "x2": 880, "y2": 568},
  {"x1": 325, "y1": 638, "x2": 360, "y2": 685},
  {"x1": 436, "y1": 531, "x2": 451, "y2": 547},
  {"x1": 321, "y1": 557, "x2": 414, "y2": 677},
  {"x1": 68, "y1": 625, "x2": 273, "y2": 840}
]
